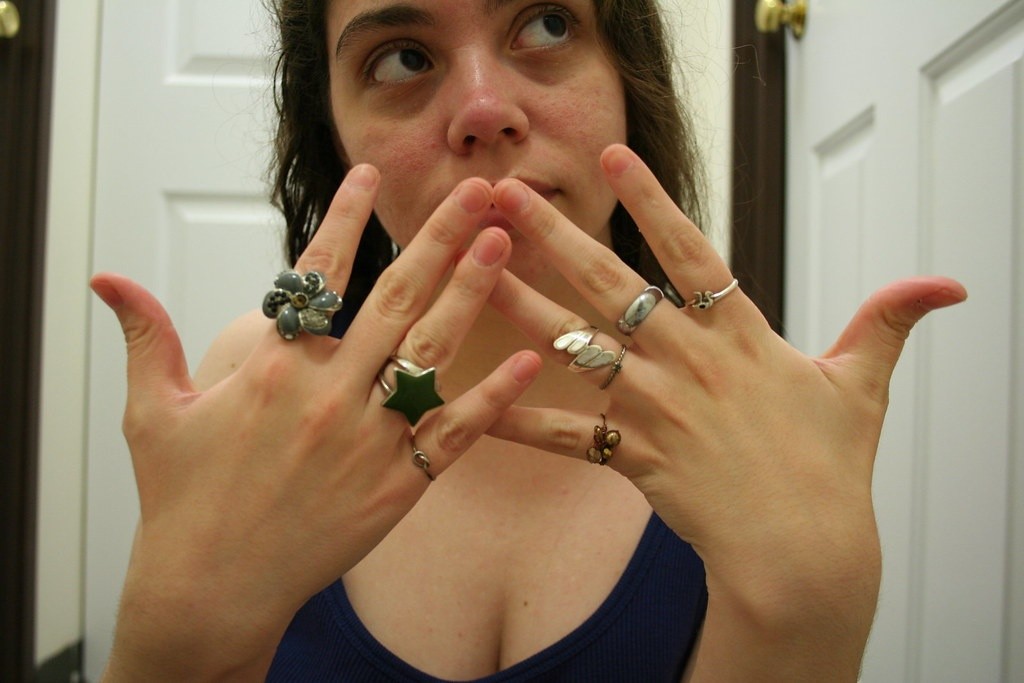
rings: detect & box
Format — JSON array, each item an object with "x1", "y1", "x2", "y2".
[
  {"x1": 587, "y1": 410, "x2": 621, "y2": 466},
  {"x1": 598, "y1": 345, "x2": 629, "y2": 390},
  {"x1": 685, "y1": 279, "x2": 738, "y2": 310},
  {"x1": 410, "y1": 435, "x2": 437, "y2": 483},
  {"x1": 552, "y1": 324, "x2": 617, "y2": 375},
  {"x1": 377, "y1": 357, "x2": 446, "y2": 427},
  {"x1": 261, "y1": 268, "x2": 344, "y2": 342},
  {"x1": 617, "y1": 287, "x2": 666, "y2": 336}
]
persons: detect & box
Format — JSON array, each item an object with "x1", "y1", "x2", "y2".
[{"x1": 86, "y1": 0, "x2": 969, "y2": 683}]
